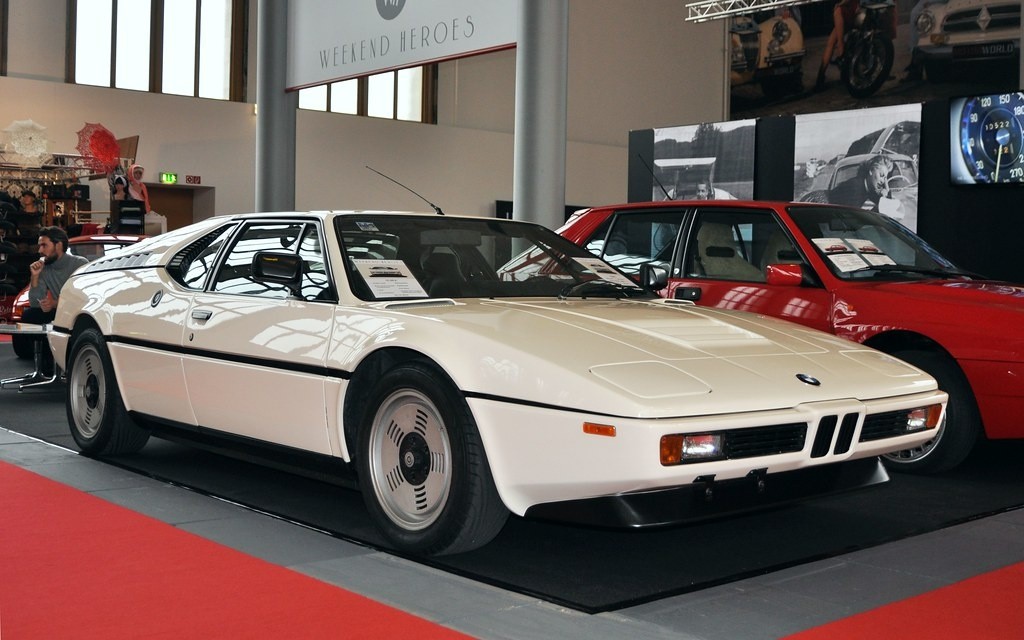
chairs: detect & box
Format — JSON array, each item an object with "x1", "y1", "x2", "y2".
[
  {"x1": 694, "y1": 223, "x2": 765, "y2": 282},
  {"x1": 761, "y1": 232, "x2": 798, "y2": 271},
  {"x1": 424, "y1": 252, "x2": 472, "y2": 297}
]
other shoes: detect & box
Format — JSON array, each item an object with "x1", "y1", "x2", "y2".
[
  {"x1": 814, "y1": 74, "x2": 826, "y2": 90},
  {"x1": 903, "y1": 64, "x2": 910, "y2": 72},
  {"x1": 899, "y1": 71, "x2": 923, "y2": 82},
  {"x1": 887, "y1": 73, "x2": 897, "y2": 80}
]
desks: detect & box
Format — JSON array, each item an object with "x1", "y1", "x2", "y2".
[{"x1": 0, "y1": 323, "x2": 67, "y2": 394}]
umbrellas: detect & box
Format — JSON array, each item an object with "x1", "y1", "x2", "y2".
[
  {"x1": 75, "y1": 122, "x2": 120, "y2": 173},
  {"x1": 0, "y1": 119, "x2": 53, "y2": 169}
]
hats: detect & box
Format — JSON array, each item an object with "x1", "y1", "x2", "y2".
[{"x1": 22, "y1": 191, "x2": 36, "y2": 198}]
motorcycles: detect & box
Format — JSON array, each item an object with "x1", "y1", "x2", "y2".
[{"x1": 838, "y1": 0, "x2": 896, "y2": 98}]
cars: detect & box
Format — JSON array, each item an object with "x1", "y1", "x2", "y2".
[
  {"x1": 12, "y1": 235, "x2": 153, "y2": 360},
  {"x1": 497, "y1": 201, "x2": 1023, "y2": 476},
  {"x1": 730, "y1": 6, "x2": 808, "y2": 101},
  {"x1": 49, "y1": 210, "x2": 949, "y2": 557},
  {"x1": 800, "y1": 122, "x2": 920, "y2": 235},
  {"x1": 915, "y1": 0, "x2": 1024, "y2": 79}
]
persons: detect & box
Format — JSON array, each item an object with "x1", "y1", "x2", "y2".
[
  {"x1": 800, "y1": 155, "x2": 896, "y2": 212},
  {"x1": 21, "y1": 226, "x2": 90, "y2": 325},
  {"x1": 696, "y1": 181, "x2": 711, "y2": 201},
  {"x1": 112, "y1": 164, "x2": 150, "y2": 213},
  {"x1": 17, "y1": 190, "x2": 41, "y2": 229}
]
